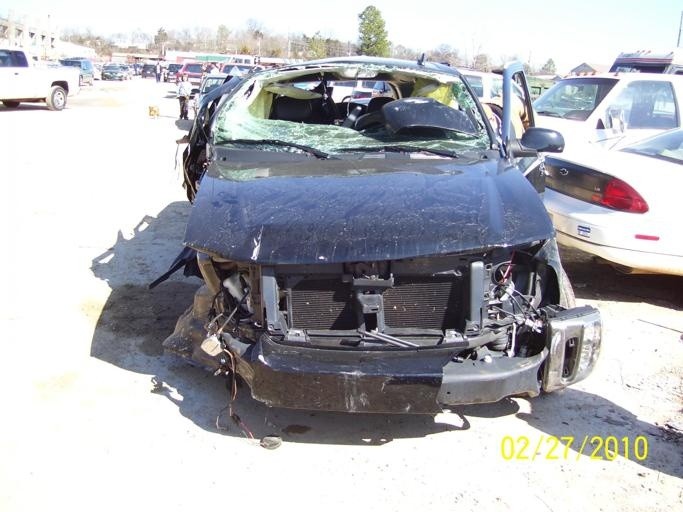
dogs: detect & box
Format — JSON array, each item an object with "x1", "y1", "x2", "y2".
[{"x1": 148, "y1": 106, "x2": 159, "y2": 117}]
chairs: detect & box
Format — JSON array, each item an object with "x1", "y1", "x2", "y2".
[
  {"x1": 271, "y1": 95, "x2": 313, "y2": 123},
  {"x1": 367, "y1": 97, "x2": 395, "y2": 111}
]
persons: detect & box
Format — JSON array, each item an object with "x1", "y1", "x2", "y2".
[
  {"x1": 175, "y1": 73, "x2": 192, "y2": 120},
  {"x1": 209, "y1": 62, "x2": 219, "y2": 74},
  {"x1": 154, "y1": 62, "x2": 162, "y2": 82},
  {"x1": 370, "y1": 80, "x2": 393, "y2": 99}
]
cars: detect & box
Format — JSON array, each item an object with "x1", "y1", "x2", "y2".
[
  {"x1": 65, "y1": 59, "x2": 94, "y2": 87},
  {"x1": 102, "y1": 63, "x2": 157, "y2": 80}
]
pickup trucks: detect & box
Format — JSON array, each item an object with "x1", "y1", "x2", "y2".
[{"x1": 0, "y1": 49, "x2": 80, "y2": 109}]
